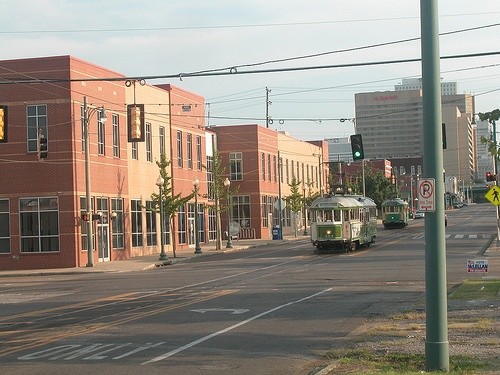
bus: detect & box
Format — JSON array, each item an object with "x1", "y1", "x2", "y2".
[
  {"x1": 380, "y1": 198, "x2": 409, "y2": 228},
  {"x1": 309, "y1": 193, "x2": 378, "y2": 253}
]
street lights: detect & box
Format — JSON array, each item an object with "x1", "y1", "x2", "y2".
[
  {"x1": 224, "y1": 177, "x2": 234, "y2": 248},
  {"x1": 192, "y1": 176, "x2": 203, "y2": 254},
  {"x1": 302, "y1": 185, "x2": 309, "y2": 235},
  {"x1": 156, "y1": 174, "x2": 169, "y2": 260}
]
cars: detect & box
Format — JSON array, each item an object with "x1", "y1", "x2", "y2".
[{"x1": 414, "y1": 210, "x2": 425, "y2": 219}]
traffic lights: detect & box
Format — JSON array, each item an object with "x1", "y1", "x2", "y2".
[
  {"x1": 82, "y1": 95, "x2": 108, "y2": 268},
  {"x1": 82, "y1": 212, "x2": 90, "y2": 221},
  {"x1": 37, "y1": 134, "x2": 48, "y2": 162},
  {"x1": 486, "y1": 171, "x2": 492, "y2": 182},
  {"x1": 350, "y1": 134, "x2": 364, "y2": 160}
]
